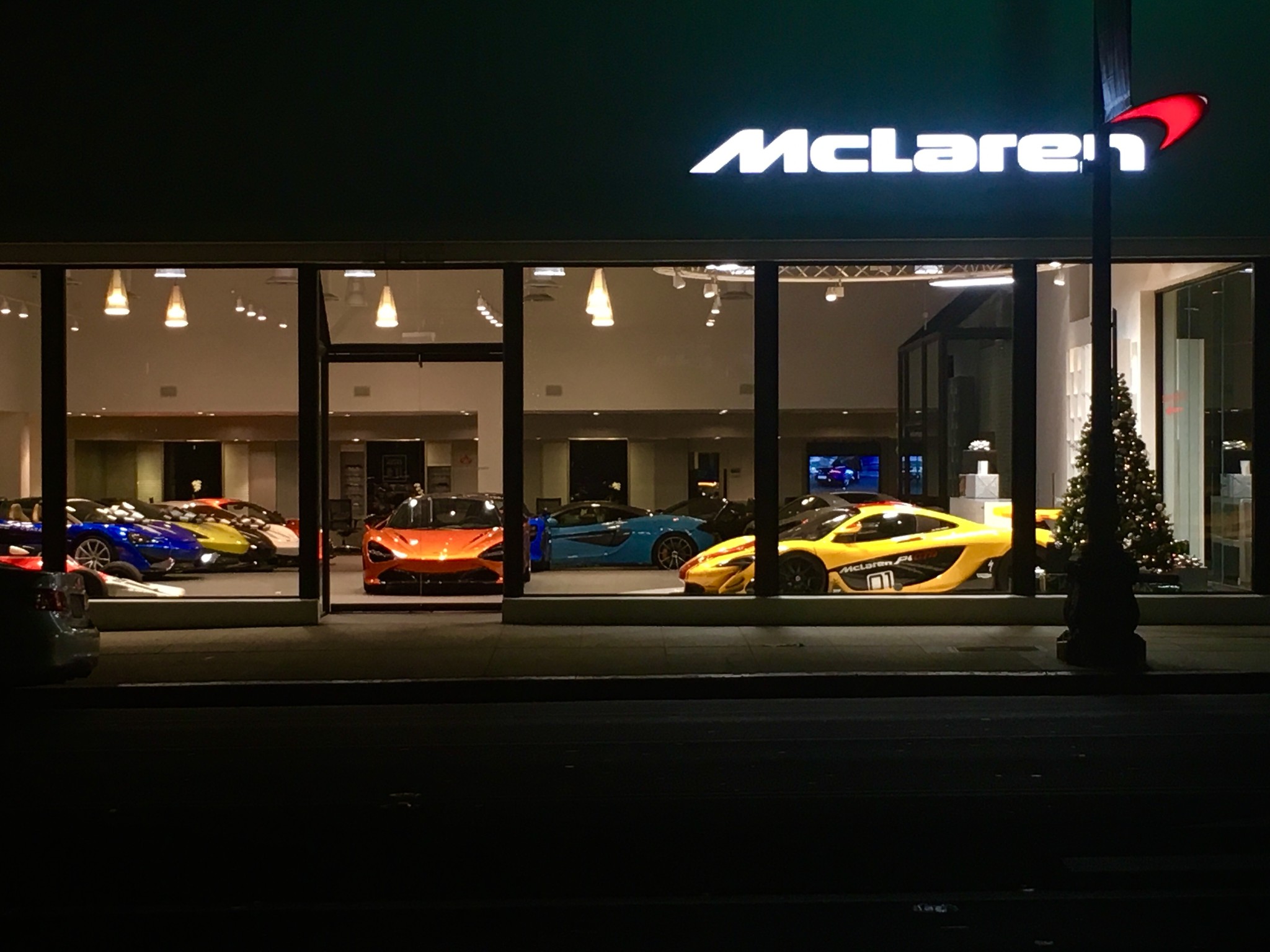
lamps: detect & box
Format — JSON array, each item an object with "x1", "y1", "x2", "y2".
[
  {"x1": 160, "y1": 282, "x2": 192, "y2": 330},
  {"x1": 582, "y1": 269, "x2": 611, "y2": 316},
  {"x1": 587, "y1": 272, "x2": 618, "y2": 326},
  {"x1": 104, "y1": 268, "x2": 134, "y2": 318},
  {"x1": 374, "y1": 283, "x2": 401, "y2": 328}
]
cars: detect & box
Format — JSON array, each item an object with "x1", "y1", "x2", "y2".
[{"x1": 2, "y1": 573, "x2": 102, "y2": 678}]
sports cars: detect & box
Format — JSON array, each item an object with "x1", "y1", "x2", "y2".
[
  {"x1": 0, "y1": 543, "x2": 105, "y2": 610},
  {"x1": 693, "y1": 503, "x2": 1061, "y2": 619},
  {"x1": 657, "y1": 498, "x2": 761, "y2": 547},
  {"x1": 201, "y1": 495, "x2": 331, "y2": 559},
  {"x1": 191, "y1": 499, "x2": 307, "y2": 559},
  {"x1": 9, "y1": 495, "x2": 207, "y2": 571},
  {"x1": 535, "y1": 500, "x2": 716, "y2": 574},
  {"x1": 1, "y1": 494, "x2": 177, "y2": 580},
  {"x1": 361, "y1": 491, "x2": 521, "y2": 601},
  {"x1": 475, "y1": 491, "x2": 555, "y2": 570},
  {"x1": 816, "y1": 463, "x2": 860, "y2": 490},
  {"x1": 147, "y1": 499, "x2": 274, "y2": 571},
  {"x1": 745, "y1": 488, "x2": 898, "y2": 538}
]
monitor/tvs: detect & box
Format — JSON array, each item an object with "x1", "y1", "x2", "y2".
[{"x1": 808, "y1": 454, "x2": 879, "y2": 496}]
